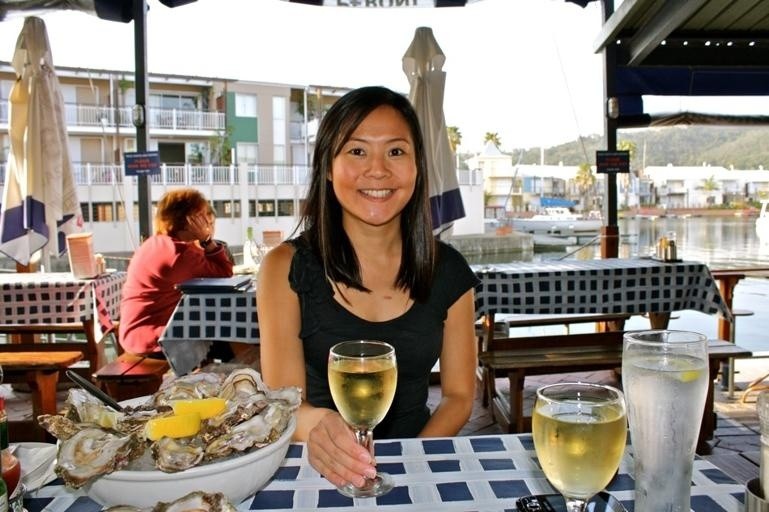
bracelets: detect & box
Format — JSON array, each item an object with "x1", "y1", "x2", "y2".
[{"x1": 198, "y1": 237, "x2": 212, "y2": 248}]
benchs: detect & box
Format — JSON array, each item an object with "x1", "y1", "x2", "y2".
[
  {"x1": 469, "y1": 312, "x2": 753, "y2": 456},
  {"x1": 0, "y1": 320, "x2": 171, "y2": 418}
]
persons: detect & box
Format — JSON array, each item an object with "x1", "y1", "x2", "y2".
[
  {"x1": 118, "y1": 189, "x2": 234, "y2": 360},
  {"x1": 202, "y1": 201, "x2": 235, "y2": 269},
  {"x1": 256, "y1": 86, "x2": 482, "y2": 487}
]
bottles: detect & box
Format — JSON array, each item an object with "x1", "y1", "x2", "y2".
[
  {"x1": 243, "y1": 227, "x2": 257, "y2": 270},
  {"x1": 660, "y1": 231, "x2": 677, "y2": 262},
  {"x1": 0, "y1": 396, "x2": 23, "y2": 512}
]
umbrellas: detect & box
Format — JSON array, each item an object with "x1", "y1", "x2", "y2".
[
  {"x1": 401, "y1": 28, "x2": 466, "y2": 241},
  {"x1": 0, "y1": 18, "x2": 88, "y2": 273}
]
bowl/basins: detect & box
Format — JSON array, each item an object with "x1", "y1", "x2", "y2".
[{"x1": 57, "y1": 394, "x2": 295, "y2": 507}]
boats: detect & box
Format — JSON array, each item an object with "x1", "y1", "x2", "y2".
[{"x1": 485, "y1": 215, "x2": 640, "y2": 252}]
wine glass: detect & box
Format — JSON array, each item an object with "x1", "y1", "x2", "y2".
[
  {"x1": 531, "y1": 382, "x2": 626, "y2": 512},
  {"x1": 328, "y1": 341, "x2": 397, "y2": 498}
]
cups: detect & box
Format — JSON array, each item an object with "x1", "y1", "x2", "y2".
[{"x1": 622, "y1": 330, "x2": 710, "y2": 512}]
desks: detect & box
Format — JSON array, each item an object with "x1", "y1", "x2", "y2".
[
  {"x1": 178, "y1": 258, "x2": 709, "y2": 425},
  {"x1": 0, "y1": 270, "x2": 127, "y2": 395}
]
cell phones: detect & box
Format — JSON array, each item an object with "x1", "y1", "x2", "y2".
[{"x1": 516, "y1": 493, "x2": 568, "y2": 512}]
